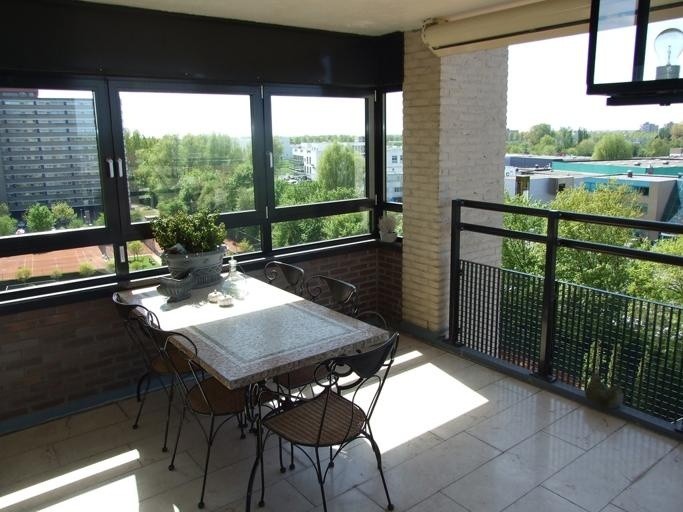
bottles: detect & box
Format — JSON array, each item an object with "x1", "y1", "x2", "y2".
[
  {"x1": 218, "y1": 251, "x2": 251, "y2": 300},
  {"x1": 173, "y1": 255, "x2": 210, "y2": 280}
]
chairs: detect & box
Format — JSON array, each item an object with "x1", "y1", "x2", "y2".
[
  {"x1": 112, "y1": 291, "x2": 203, "y2": 454},
  {"x1": 264, "y1": 261, "x2": 360, "y2": 390},
  {"x1": 246, "y1": 330, "x2": 402, "y2": 512},
  {"x1": 133, "y1": 310, "x2": 257, "y2": 509}
]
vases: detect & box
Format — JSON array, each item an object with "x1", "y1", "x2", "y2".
[{"x1": 379, "y1": 230, "x2": 397, "y2": 243}]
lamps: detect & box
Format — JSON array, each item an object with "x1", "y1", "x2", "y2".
[{"x1": 585, "y1": 0, "x2": 683, "y2": 108}]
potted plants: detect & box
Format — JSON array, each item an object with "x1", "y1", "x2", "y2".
[{"x1": 149, "y1": 206, "x2": 228, "y2": 289}]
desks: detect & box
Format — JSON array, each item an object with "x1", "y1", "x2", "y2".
[{"x1": 116, "y1": 270, "x2": 391, "y2": 512}]
